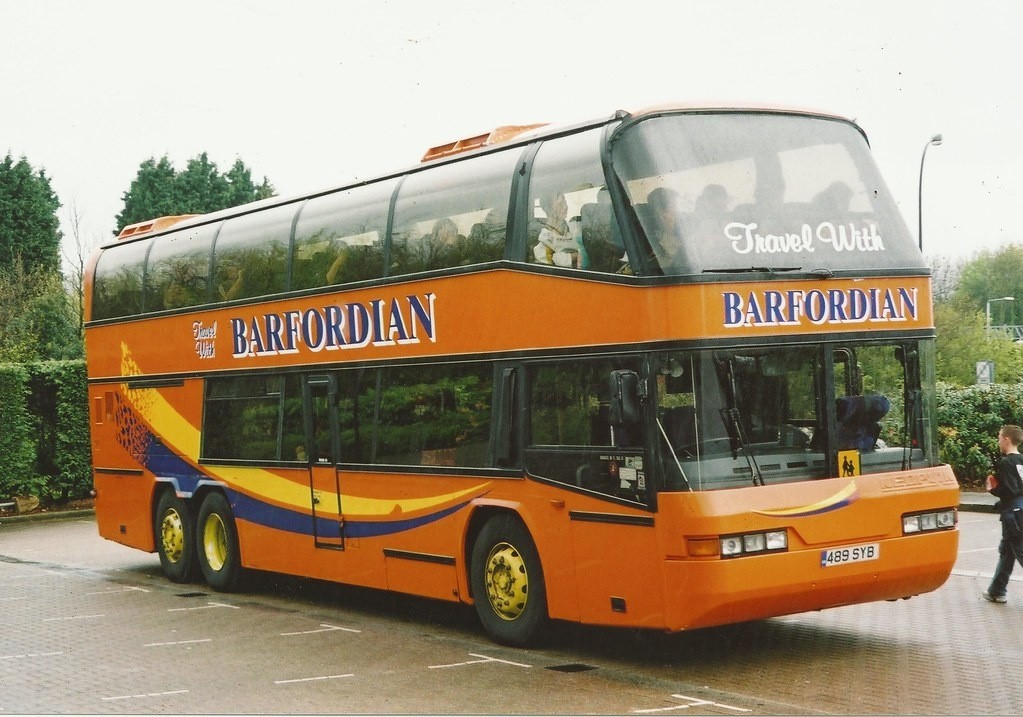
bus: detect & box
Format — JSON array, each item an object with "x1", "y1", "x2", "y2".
[{"x1": 82, "y1": 108, "x2": 959, "y2": 651}]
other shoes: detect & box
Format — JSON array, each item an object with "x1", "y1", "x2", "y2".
[{"x1": 982, "y1": 592, "x2": 1008, "y2": 603}]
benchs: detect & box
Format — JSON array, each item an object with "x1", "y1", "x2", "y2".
[{"x1": 109, "y1": 202, "x2": 814, "y2": 320}]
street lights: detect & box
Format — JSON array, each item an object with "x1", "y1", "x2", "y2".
[
  {"x1": 917, "y1": 134, "x2": 944, "y2": 253},
  {"x1": 986, "y1": 297, "x2": 1015, "y2": 338}
]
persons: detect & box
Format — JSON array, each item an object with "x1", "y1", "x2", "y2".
[
  {"x1": 231, "y1": 397, "x2": 308, "y2": 463},
  {"x1": 981, "y1": 424, "x2": 1023, "y2": 603},
  {"x1": 93, "y1": 178, "x2": 885, "y2": 319}
]
